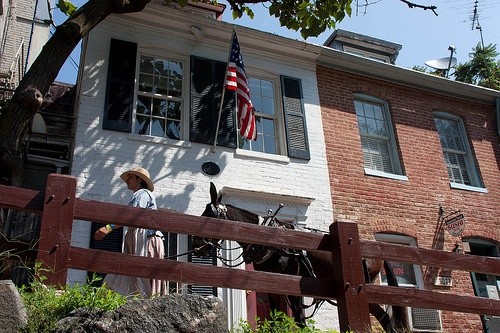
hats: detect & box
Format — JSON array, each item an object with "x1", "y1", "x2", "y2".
[{"x1": 119, "y1": 167, "x2": 155, "y2": 192}]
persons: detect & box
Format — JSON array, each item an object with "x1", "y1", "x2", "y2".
[{"x1": 94, "y1": 167, "x2": 167, "y2": 300}]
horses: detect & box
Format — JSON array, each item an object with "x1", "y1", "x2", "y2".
[{"x1": 190, "y1": 181, "x2": 411, "y2": 333}]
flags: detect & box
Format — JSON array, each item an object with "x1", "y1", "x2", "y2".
[{"x1": 224, "y1": 28, "x2": 257, "y2": 141}]
